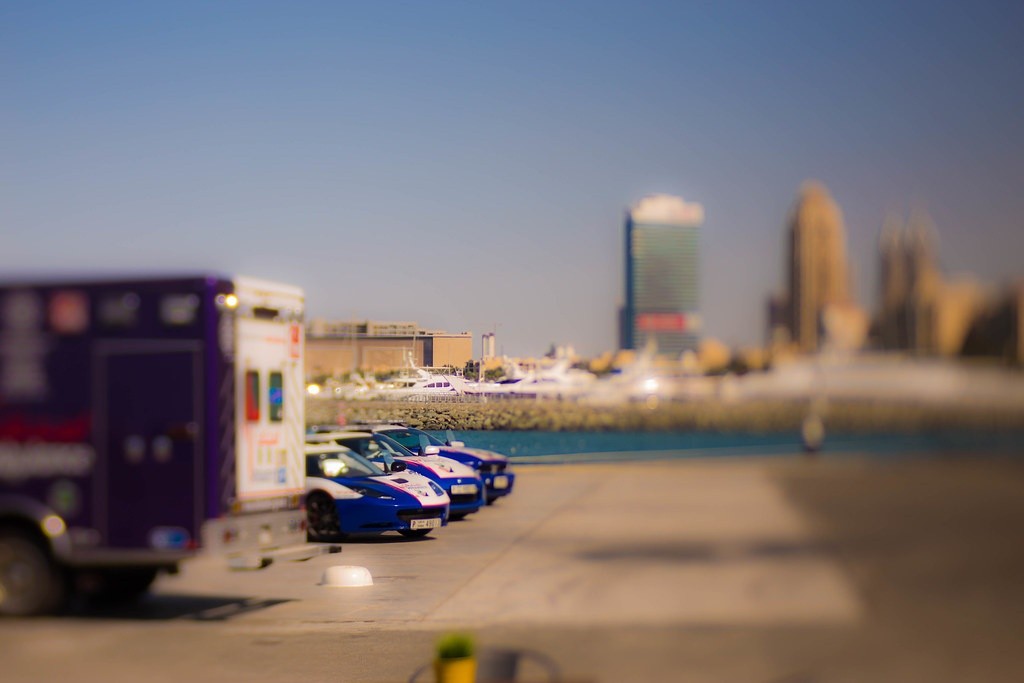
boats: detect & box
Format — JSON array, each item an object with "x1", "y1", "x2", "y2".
[{"x1": 379, "y1": 370, "x2": 499, "y2": 397}]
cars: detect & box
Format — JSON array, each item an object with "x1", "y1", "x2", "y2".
[
  {"x1": 304, "y1": 443, "x2": 451, "y2": 543},
  {"x1": 304, "y1": 433, "x2": 486, "y2": 521},
  {"x1": 304, "y1": 423, "x2": 514, "y2": 509}
]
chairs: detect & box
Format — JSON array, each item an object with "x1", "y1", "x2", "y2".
[{"x1": 407, "y1": 644, "x2": 561, "y2": 682}]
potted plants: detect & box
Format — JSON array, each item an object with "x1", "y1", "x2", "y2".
[{"x1": 430, "y1": 631, "x2": 481, "y2": 683}]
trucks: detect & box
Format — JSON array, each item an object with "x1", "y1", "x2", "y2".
[{"x1": 0, "y1": 268, "x2": 343, "y2": 619}]
